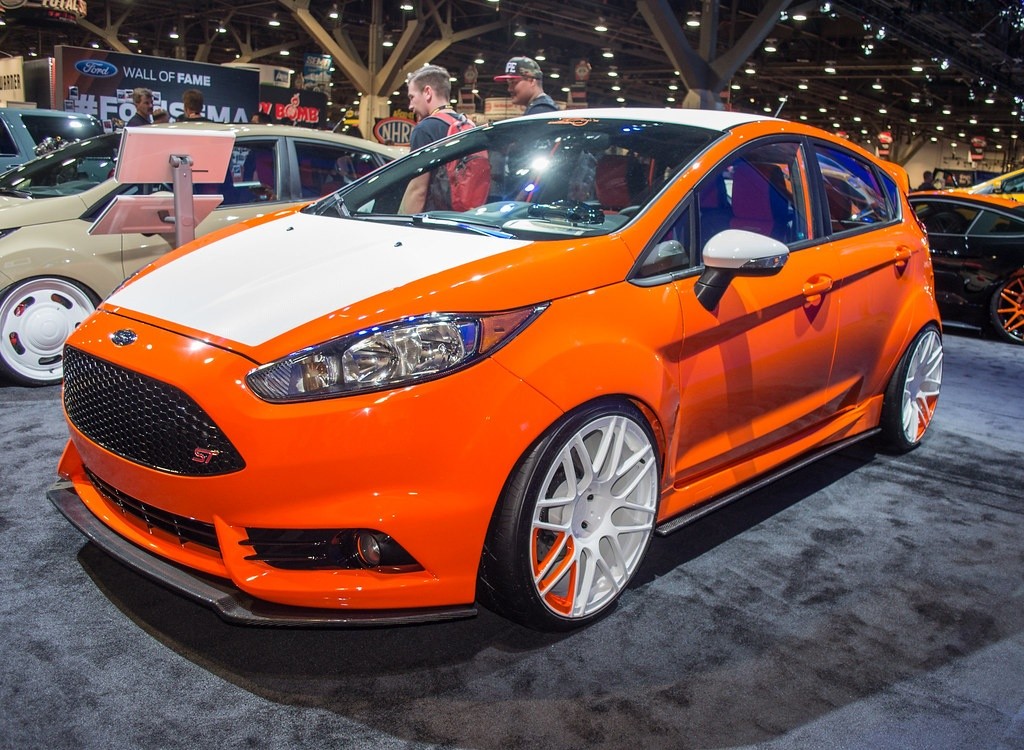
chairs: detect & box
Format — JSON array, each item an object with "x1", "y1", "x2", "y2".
[
  {"x1": 696, "y1": 162, "x2": 790, "y2": 253},
  {"x1": 592, "y1": 156, "x2": 644, "y2": 218}
]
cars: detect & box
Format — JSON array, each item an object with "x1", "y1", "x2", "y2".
[
  {"x1": 726, "y1": 162, "x2": 886, "y2": 234},
  {"x1": 908, "y1": 192, "x2": 1024, "y2": 349},
  {"x1": 0, "y1": 121, "x2": 411, "y2": 391},
  {"x1": 45, "y1": 109, "x2": 945, "y2": 634},
  {"x1": 945, "y1": 168, "x2": 1024, "y2": 203}
]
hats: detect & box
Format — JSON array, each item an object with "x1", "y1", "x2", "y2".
[{"x1": 493, "y1": 56, "x2": 543, "y2": 82}]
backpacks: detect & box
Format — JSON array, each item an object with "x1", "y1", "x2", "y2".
[{"x1": 423, "y1": 113, "x2": 492, "y2": 211}]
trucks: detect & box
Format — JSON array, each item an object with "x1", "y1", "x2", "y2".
[{"x1": 0, "y1": 106, "x2": 102, "y2": 181}]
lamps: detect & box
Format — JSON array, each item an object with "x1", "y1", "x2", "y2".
[{"x1": 0, "y1": 0, "x2": 1024, "y2": 171}]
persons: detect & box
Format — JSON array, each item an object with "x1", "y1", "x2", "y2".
[
  {"x1": 494, "y1": 56, "x2": 574, "y2": 203},
  {"x1": 395, "y1": 65, "x2": 467, "y2": 217},
  {"x1": 127, "y1": 88, "x2": 375, "y2": 206},
  {"x1": 918, "y1": 171, "x2": 938, "y2": 191}
]
